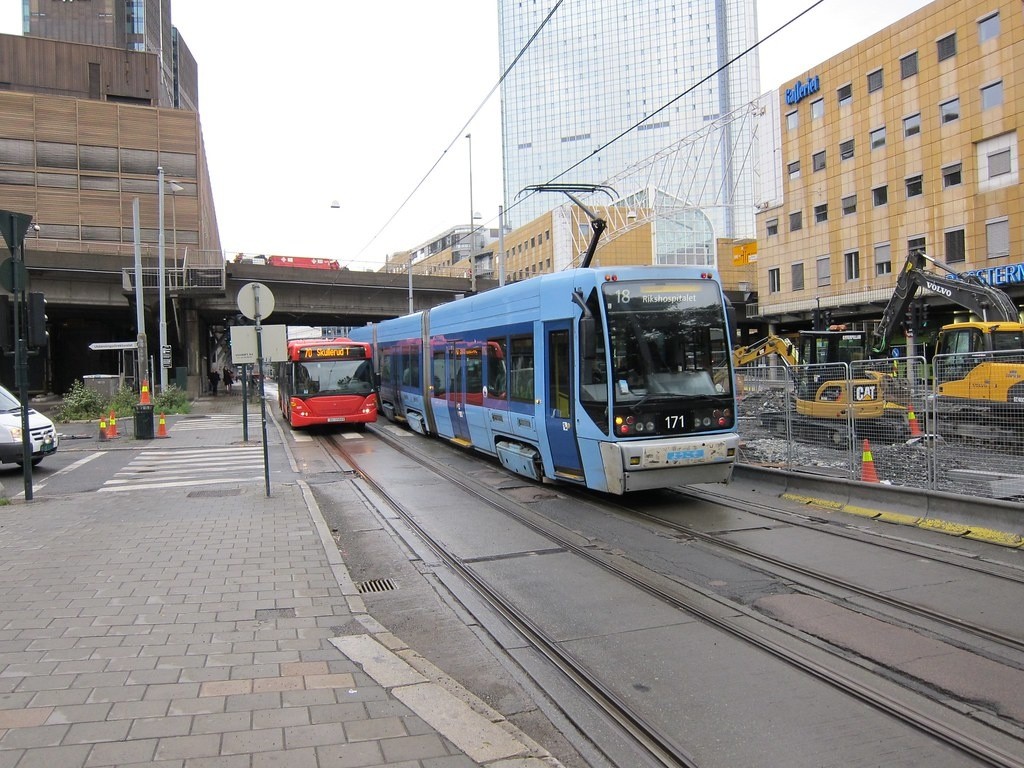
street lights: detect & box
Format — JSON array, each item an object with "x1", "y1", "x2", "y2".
[
  {"x1": 466, "y1": 132, "x2": 477, "y2": 294},
  {"x1": 159, "y1": 165, "x2": 182, "y2": 394}
]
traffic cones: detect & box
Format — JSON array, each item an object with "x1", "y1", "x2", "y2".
[
  {"x1": 907, "y1": 406, "x2": 925, "y2": 439},
  {"x1": 139, "y1": 379, "x2": 150, "y2": 405},
  {"x1": 107, "y1": 409, "x2": 119, "y2": 439},
  {"x1": 98, "y1": 415, "x2": 109, "y2": 441},
  {"x1": 860, "y1": 439, "x2": 880, "y2": 483},
  {"x1": 155, "y1": 412, "x2": 170, "y2": 438}
]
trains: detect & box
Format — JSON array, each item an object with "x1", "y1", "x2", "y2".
[{"x1": 347, "y1": 263, "x2": 740, "y2": 496}]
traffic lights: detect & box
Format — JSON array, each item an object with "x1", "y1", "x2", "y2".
[{"x1": 29, "y1": 295, "x2": 50, "y2": 347}]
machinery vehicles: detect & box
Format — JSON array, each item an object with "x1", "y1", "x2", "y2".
[
  {"x1": 711, "y1": 333, "x2": 910, "y2": 450},
  {"x1": 865, "y1": 247, "x2": 1023, "y2": 440}
]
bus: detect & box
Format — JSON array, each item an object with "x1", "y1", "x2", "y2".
[{"x1": 278, "y1": 336, "x2": 378, "y2": 431}]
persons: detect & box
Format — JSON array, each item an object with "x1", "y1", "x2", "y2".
[
  {"x1": 208, "y1": 366, "x2": 239, "y2": 397},
  {"x1": 634, "y1": 341, "x2": 673, "y2": 376}
]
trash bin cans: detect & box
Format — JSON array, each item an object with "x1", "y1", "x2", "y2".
[{"x1": 132, "y1": 404, "x2": 154, "y2": 439}]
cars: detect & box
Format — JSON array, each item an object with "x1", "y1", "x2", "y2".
[{"x1": 0, "y1": 384, "x2": 58, "y2": 470}]
